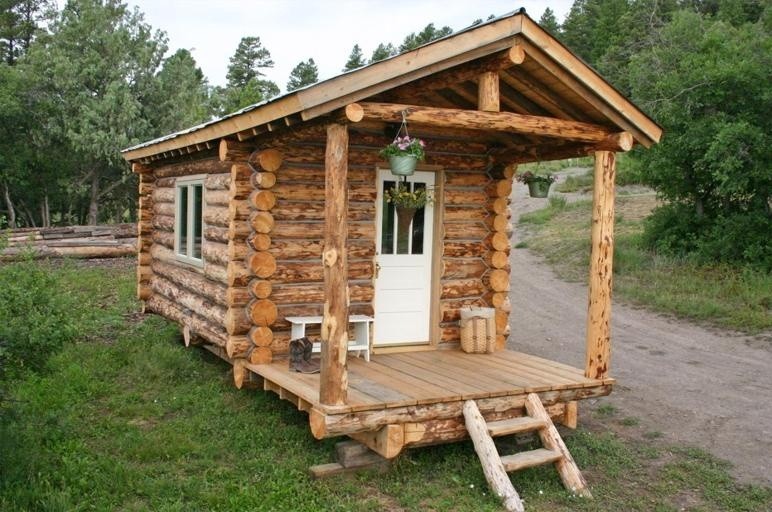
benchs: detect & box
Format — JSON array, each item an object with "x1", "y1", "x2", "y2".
[{"x1": 285, "y1": 314, "x2": 375, "y2": 362}]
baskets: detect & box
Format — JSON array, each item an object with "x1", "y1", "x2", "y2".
[{"x1": 459, "y1": 316, "x2": 496, "y2": 354}]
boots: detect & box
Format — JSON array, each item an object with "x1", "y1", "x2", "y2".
[{"x1": 289, "y1": 338, "x2": 321, "y2": 373}]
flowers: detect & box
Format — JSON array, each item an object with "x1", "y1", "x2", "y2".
[
  {"x1": 515, "y1": 170, "x2": 560, "y2": 191},
  {"x1": 378, "y1": 135, "x2": 428, "y2": 163},
  {"x1": 384, "y1": 181, "x2": 433, "y2": 207}
]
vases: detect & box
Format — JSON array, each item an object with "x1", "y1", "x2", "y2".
[
  {"x1": 397, "y1": 206, "x2": 418, "y2": 229},
  {"x1": 387, "y1": 153, "x2": 417, "y2": 176},
  {"x1": 526, "y1": 180, "x2": 551, "y2": 198}
]
